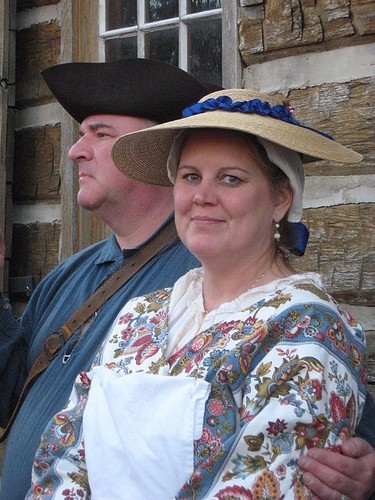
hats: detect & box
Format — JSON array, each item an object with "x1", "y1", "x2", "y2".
[
  {"x1": 40, "y1": 58, "x2": 228, "y2": 125},
  {"x1": 110, "y1": 88, "x2": 364, "y2": 187}
]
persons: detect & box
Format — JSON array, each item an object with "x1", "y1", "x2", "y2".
[
  {"x1": 23, "y1": 87, "x2": 364, "y2": 500},
  {"x1": 0, "y1": 58, "x2": 375, "y2": 500}
]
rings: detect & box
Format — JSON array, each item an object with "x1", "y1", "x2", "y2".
[{"x1": 341, "y1": 494, "x2": 346, "y2": 500}]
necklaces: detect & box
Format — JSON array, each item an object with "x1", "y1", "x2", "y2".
[{"x1": 236, "y1": 251, "x2": 276, "y2": 295}]
